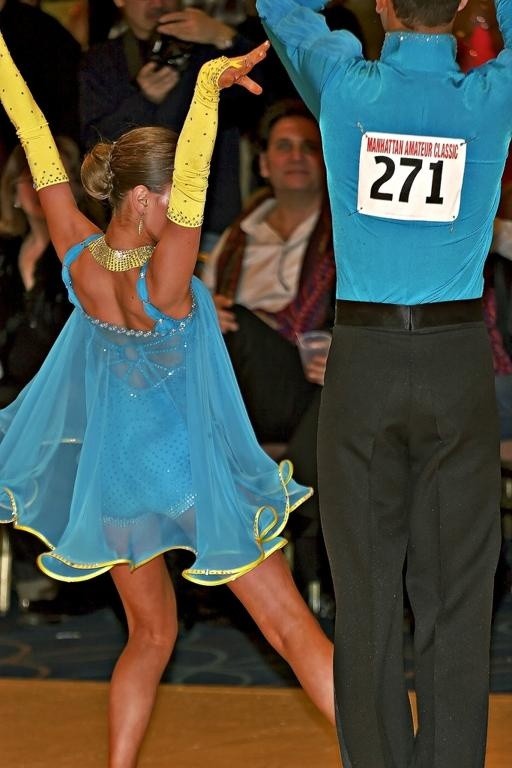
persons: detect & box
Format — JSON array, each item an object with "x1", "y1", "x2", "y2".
[
  {"x1": 252, "y1": 0, "x2": 510, "y2": 768},
  {"x1": 2, "y1": 28, "x2": 336, "y2": 768}
]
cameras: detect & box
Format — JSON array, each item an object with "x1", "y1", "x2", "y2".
[{"x1": 151, "y1": 26, "x2": 193, "y2": 75}]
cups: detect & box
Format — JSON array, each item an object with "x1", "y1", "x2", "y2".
[{"x1": 294, "y1": 332, "x2": 333, "y2": 382}]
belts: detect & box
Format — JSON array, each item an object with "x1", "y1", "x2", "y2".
[{"x1": 334, "y1": 298, "x2": 487, "y2": 332}]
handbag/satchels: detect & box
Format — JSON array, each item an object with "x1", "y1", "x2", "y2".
[{"x1": 219, "y1": 304, "x2": 315, "y2": 445}]
